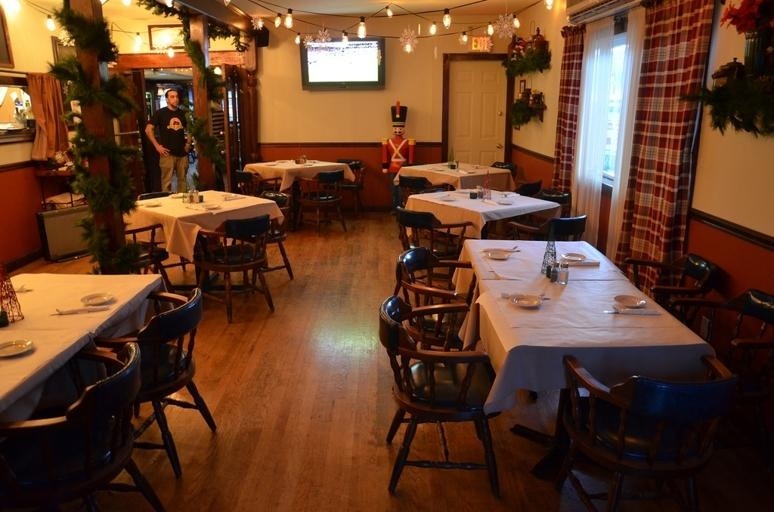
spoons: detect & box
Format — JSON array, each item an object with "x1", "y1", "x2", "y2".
[{"x1": 507, "y1": 246, "x2": 522, "y2": 255}]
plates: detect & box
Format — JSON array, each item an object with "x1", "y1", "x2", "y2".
[
  {"x1": 564, "y1": 253, "x2": 587, "y2": 261},
  {"x1": 439, "y1": 195, "x2": 455, "y2": 201},
  {"x1": 499, "y1": 200, "x2": 513, "y2": 204},
  {"x1": 80, "y1": 292, "x2": 112, "y2": 306},
  {"x1": 143, "y1": 201, "x2": 163, "y2": 207},
  {"x1": 203, "y1": 203, "x2": 219, "y2": 209},
  {"x1": 511, "y1": 294, "x2": 542, "y2": 308},
  {"x1": 614, "y1": 294, "x2": 647, "y2": 308},
  {"x1": 0, "y1": 339, "x2": 33, "y2": 358},
  {"x1": 487, "y1": 248, "x2": 511, "y2": 259}
]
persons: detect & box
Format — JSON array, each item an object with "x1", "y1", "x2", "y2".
[
  {"x1": 144, "y1": 90, "x2": 192, "y2": 193},
  {"x1": 379, "y1": 101, "x2": 416, "y2": 216}
]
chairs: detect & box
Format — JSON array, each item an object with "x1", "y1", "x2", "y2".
[
  {"x1": 230, "y1": 190, "x2": 295, "y2": 286},
  {"x1": 395, "y1": 174, "x2": 456, "y2": 242},
  {"x1": 534, "y1": 188, "x2": 570, "y2": 218},
  {"x1": 668, "y1": 287, "x2": 774, "y2": 387},
  {"x1": 552, "y1": 353, "x2": 739, "y2": 511},
  {"x1": 395, "y1": 204, "x2": 472, "y2": 290},
  {"x1": 192, "y1": 214, "x2": 275, "y2": 324},
  {"x1": 136, "y1": 191, "x2": 186, "y2": 275},
  {"x1": 295, "y1": 169, "x2": 347, "y2": 238},
  {"x1": 323, "y1": 160, "x2": 368, "y2": 225},
  {"x1": 92, "y1": 287, "x2": 217, "y2": 480},
  {"x1": 491, "y1": 160, "x2": 515, "y2": 177},
  {"x1": 322, "y1": 159, "x2": 353, "y2": 215},
  {"x1": 0, "y1": 341, "x2": 164, "y2": 512},
  {"x1": 377, "y1": 294, "x2": 503, "y2": 500},
  {"x1": 623, "y1": 253, "x2": 722, "y2": 342},
  {"x1": 393, "y1": 246, "x2": 476, "y2": 372},
  {"x1": 234, "y1": 169, "x2": 283, "y2": 197},
  {"x1": 487, "y1": 177, "x2": 543, "y2": 198},
  {"x1": 506, "y1": 212, "x2": 588, "y2": 242},
  {"x1": 123, "y1": 221, "x2": 175, "y2": 294}
]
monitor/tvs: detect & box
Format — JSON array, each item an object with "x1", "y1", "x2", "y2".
[{"x1": 299, "y1": 36, "x2": 386, "y2": 90}]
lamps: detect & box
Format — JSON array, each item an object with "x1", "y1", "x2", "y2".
[
  {"x1": 341, "y1": 28, "x2": 345, "y2": 36},
  {"x1": 47, "y1": 14, "x2": 55, "y2": 32},
  {"x1": 385, "y1": 6, "x2": 393, "y2": 18},
  {"x1": 295, "y1": 32, "x2": 301, "y2": 45},
  {"x1": 133, "y1": 32, "x2": 143, "y2": 52},
  {"x1": 513, "y1": 14, "x2": 520, "y2": 30},
  {"x1": 429, "y1": 21, "x2": 437, "y2": 36},
  {"x1": 274, "y1": 13, "x2": 281, "y2": 28},
  {"x1": 443, "y1": 10, "x2": 451, "y2": 30},
  {"x1": 342, "y1": 33, "x2": 349, "y2": 42},
  {"x1": 462, "y1": 32, "x2": 468, "y2": 42},
  {"x1": 284, "y1": 8, "x2": 293, "y2": 29},
  {"x1": 357, "y1": 15, "x2": 367, "y2": 38}
]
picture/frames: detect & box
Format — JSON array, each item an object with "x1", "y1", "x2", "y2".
[{"x1": 147, "y1": 24, "x2": 185, "y2": 49}]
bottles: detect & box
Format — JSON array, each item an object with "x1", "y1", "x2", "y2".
[
  {"x1": 551, "y1": 262, "x2": 560, "y2": 281},
  {"x1": 193, "y1": 189, "x2": 199, "y2": 203},
  {"x1": 478, "y1": 187, "x2": 483, "y2": 199},
  {"x1": 301, "y1": 151, "x2": 307, "y2": 163},
  {"x1": 187, "y1": 189, "x2": 194, "y2": 203},
  {"x1": 558, "y1": 262, "x2": 569, "y2": 285}
]
